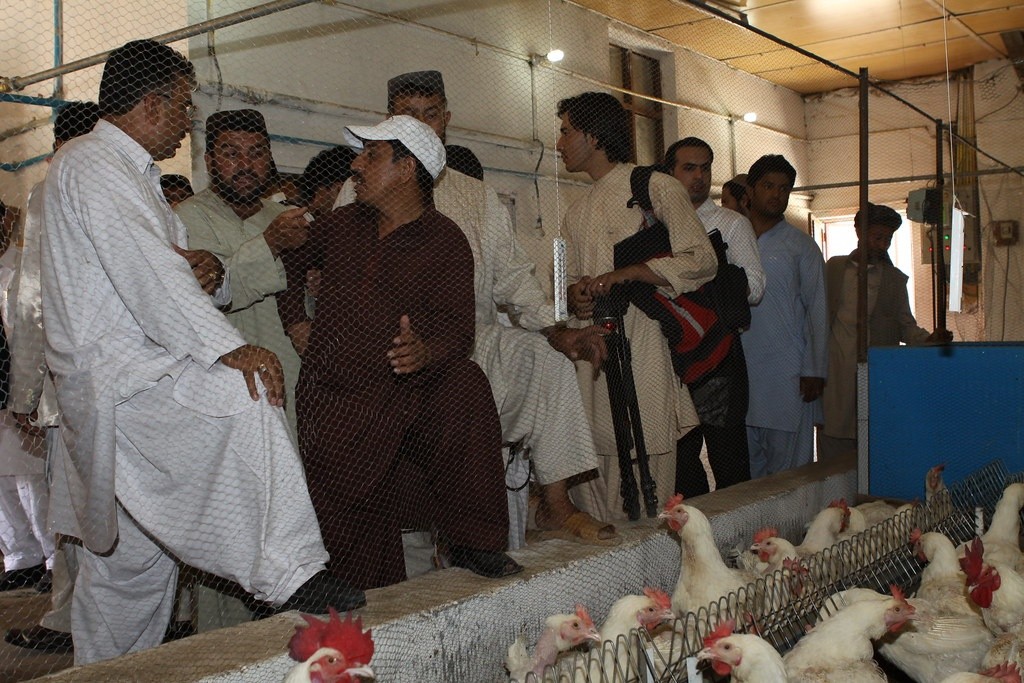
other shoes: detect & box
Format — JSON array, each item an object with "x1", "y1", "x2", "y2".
[
  {"x1": 163, "y1": 620, "x2": 196, "y2": 642},
  {"x1": 0, "y1": 564, "x2": 53, "y2": 593}
]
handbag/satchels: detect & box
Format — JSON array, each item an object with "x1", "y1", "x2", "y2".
[{"x1": 612, "y1": 224, "x2": 741, "y2": 387}]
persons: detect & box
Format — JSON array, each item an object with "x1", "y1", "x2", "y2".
[
  {"x1": 275, "y1": 114, "x2": 524, "y2": 592},
  {"x1": 39, "y1": 39, "x2": 366, "y2": 666},
  {"x1": 159, "y1": 145, "x2": 485, "y2": 209},
  {"x1": 330, "y1": 70, "x2": 623, "y2": 578},
  {"x1": 163, "y1": 109, "x2": 320, "y2": 644},
  {"x1": 544, "y1": 92, "x2": 719, "y2": 525},
  {"x1": 816, "y1": 202, "x2": 954, "y2": 459},
  {"x1": 663, "y1": 136, "x2": 767, "y2": 501},
  {"x1": 0, "y1": 99, "x2": 101, "y2": 652},
  {"x1": 722, "y1": 155, "x2": 830, "y2": 479}
]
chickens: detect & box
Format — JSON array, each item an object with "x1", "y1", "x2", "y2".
[
  {"x1": 280, "y1": 606, "x2": 377, "y2": 683},
  {"x1": 505, "y1": 463, "x2": 1024, "y2": 683}
]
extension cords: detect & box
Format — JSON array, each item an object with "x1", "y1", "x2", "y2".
[{"x1": 553, "y1": 238, "x2": 567, "y2": 322}]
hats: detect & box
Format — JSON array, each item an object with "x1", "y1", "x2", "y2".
[
  {"x1": 855, "y1": 203, "x2": 902, "y2": 231},
  {"x1": 388, "y1": 70, "x2": 446, "y2": 107},
  {"x1": 206, "y1": 109, "x2": 267, "y2": 144},
  {"x1": 343, "y1": 115, "x2": 447, "y2": 180},
  {"x1": 733, "y1": 173, "x2": 749, "y2": 187}
]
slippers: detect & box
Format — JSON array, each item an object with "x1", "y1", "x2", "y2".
[{"x1": 526, "y1": 509, "x2": 623, "y2": 547}]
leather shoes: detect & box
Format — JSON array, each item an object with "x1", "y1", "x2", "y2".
[
  {"x1": 3, "y1": 625, "x2": 74, "y2": 652},
  {"x1": 447, "y1": 543, "x2": 521, "y2": 578},
  {"x1": 286, "y1": 570, "x2": 367, "y2": 614}
]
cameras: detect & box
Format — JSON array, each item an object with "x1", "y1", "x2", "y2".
[{"x1": 581, "y1": 283, "x2": 630, "y2": 317}]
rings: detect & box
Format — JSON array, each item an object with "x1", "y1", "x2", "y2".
[
  {"x1": 310, "y1": 276, "x2": 315, "y2": 280},
  {"x1": 599, "y1": 282, "x2": 605, "y2": 286},
  {"x1": 209, "y1": 270, "x2": 225, "y2": 281},
  {"x1": 257, "y1": 363, "x2": 267, "y2": 374}
]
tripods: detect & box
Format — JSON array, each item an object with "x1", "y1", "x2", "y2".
[{"x1": 592, "y1": 318, "x2": 659, "y2": 521}]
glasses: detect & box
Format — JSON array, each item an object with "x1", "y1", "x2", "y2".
[{"x1": 154, "y1": 92, "x2": 199, "y2": 117}]
